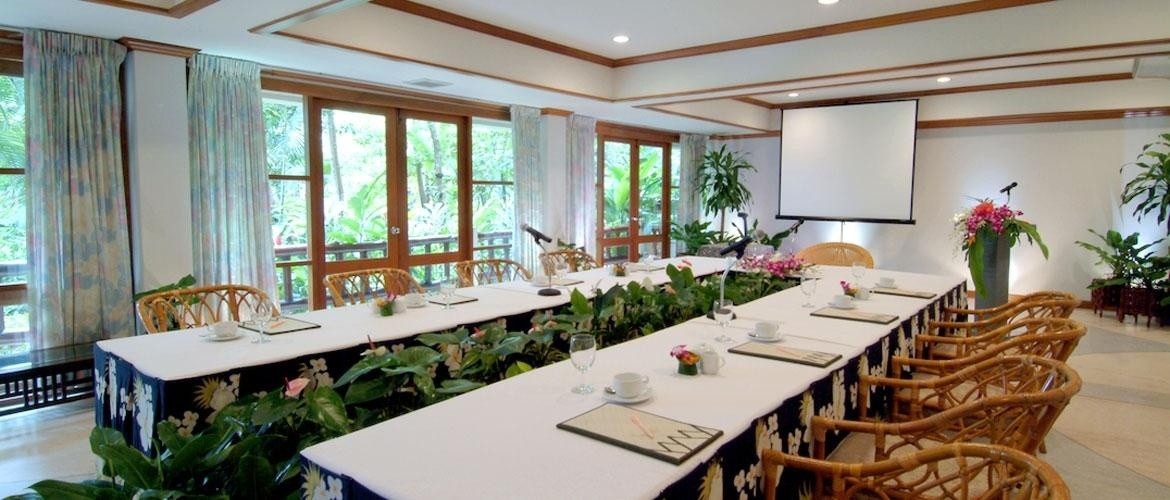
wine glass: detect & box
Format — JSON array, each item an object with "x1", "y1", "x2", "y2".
[
  {"x1": 801, "y1": 275, "x2": 816, "y2": 308},
  {"x1": 853, "y1": 261, "x2": 865, "y2": 284},
  {"x1": 556, "y1": 262, "x2": 568, "y2": 291},
  {"x1": 643, "y1": 252, "x2": 654, "y2": 278},
  {"x1": 570, "y1": 333, "x2": 597, "y2": 395},
  {"x1": 249, "y1": 298, "x2": 273, "y2": 344},
  {"x1": 716, "y1": 300, "x2": 733, "y2": 341},
  {"x1": 440, "y1": 275, "x2": 455, "y2": 311}
]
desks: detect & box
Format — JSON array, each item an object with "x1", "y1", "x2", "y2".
[{"x1": 92, "y1": 254, "x2": 970, "y2": 500}]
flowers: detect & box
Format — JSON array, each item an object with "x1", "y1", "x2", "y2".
[
  {"x1": 732, "y1": 255, "x2": 817, "y2": 303},
  {"x1": 950, "y1": 195, "x2": 1048, "y2": 298},
  {"x1": 528, "y1": 261, "x2": 712, "y2": 363},
  {"x1": 377, "y1": 298, "x2": 394, "y2": 308},
  {"x1": 840, "y1": 281, "x2": 858, "y2": 293},
  {"x1": 249, "y1": 376, "x2": 352, "y2": 448},
  {"x1": 334, "y1": 335, "x2": 480, "y2": 421},
  {"x1": 412, "y1": 318, "x2": 532, "y2": 385}
]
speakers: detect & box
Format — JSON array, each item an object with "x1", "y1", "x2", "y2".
[{"x1": 1131, "y1": 55, "x2": 1170, "y2": 81}]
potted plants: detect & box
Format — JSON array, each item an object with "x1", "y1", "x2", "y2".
[
  {"x1": 1087, "y1": 250, "x2": 1170, "y2": 327},
  {"x1": 1076, "y1": 228, "x2": 1140, "y2": 317}
]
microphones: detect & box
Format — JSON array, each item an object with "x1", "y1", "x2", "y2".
[
  {"x1": 737, "y1": 213, "x2": 748, "y2": 217},
  {"x1": 1000, "y1": 182, "x2": 1018, "y2": 193},
  {"x1": 789, "y1": 217, "x2": 805, "y2": 231},
  {"x1": 720, "y1": 236, "x2": 752, "y2": 255},
  {"x1": 519, "y1": 222, "x2": 552, "y2": 243}
]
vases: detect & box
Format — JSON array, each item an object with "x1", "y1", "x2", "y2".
[
  {"x1": 678, "y1": 362, "x2": 698, "y2": 376},
  {"x1": 844, "y1": 293, "x2": 855, "y2": 298},
  {"x1": 380, "y1": 305, "x2": 393, "y2": 317}
]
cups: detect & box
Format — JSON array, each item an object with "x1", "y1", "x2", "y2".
[
  {"x1": 407, "y1": 293, "x2": 422, "y2": 302},
  {"x1": 879, "y1": 277, "x2": 894, "y2": 286},
  {"x1": 612, "y1": 371, "x2": 651, "y2": 397},
  {"x1": 756, "y1": 321, "x2": 781, "y2": 337},
  {"x1": 535, "y1": 276, "x2": 546, "y2": 282},
  {"x1": 214, "y1": 322, "x2": 239, "y2": 336},
  {"x1": 700, "y1": 347, "x2": 726, "y2": 373},
  {"x1": 833, "y1": 295, "x2": 856, "y2": 304},
  {"x1": 628, "y1": 264, "x2": 639, "y2": 272}
]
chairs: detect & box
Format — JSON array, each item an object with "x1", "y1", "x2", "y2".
[
  {"x1": 927, "y1": 290, "x2": 1082, "y2": 361},
  {"x1": 810, "y1": 355, "x2": 1083, "y2": 500},
  {"x1": 455, "y1": 259, "x2": 531, "y2": 288},
  {"x1": 892, "y1": 318, "x2": 1088, "y2": 454},
  {"x1": 135, "y1": 285, "x2": 280, "y2": 334},
  {"x1": 540, "y1": 249, "x2": 600, "y2": 275},
  {"x1": 323, "y1": 267, "x2": 425, "y2": 308},
  {"x1": 795, "y1": 242, "x2": 875, "y2": 269},
  {"x1": 696, "y1": 242, "x2": 774, "y2": 258},
  {"x1": 762, "y1": 443, "x2": 1069, "y2": 500}
]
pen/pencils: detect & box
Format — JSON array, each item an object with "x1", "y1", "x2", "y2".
[
  {"x1": 775, "y1": 343, "x2": 803, "y2": 359},
  {"x1": 269, "y1": 317, "x2": 290, "y2": 331},
  {"x1": 630, "y1": 413, "x2": 654, "y2": 443}
]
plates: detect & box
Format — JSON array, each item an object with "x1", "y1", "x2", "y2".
[
  {"x1": 874, "y1": 282, "x2": 901, "y2": 290},
  {"x1": 827, "y1": 300, "x2": 859, "y2": 309},
  {"x1": 747, "y1": 331, "x2": 784, "y2": 342},
  {"x1": 533, "y1": 283, "x2": 548, "y2": 286},
  {"x1": 602, "y1": 387, "x2": 655, "y2": 404},
  {"x1": 406, "y1": 299, "x2": 430, "y2": 309},
  {"x1": 207, "y1": 331, "x2": 246, "y2": 341}
]
